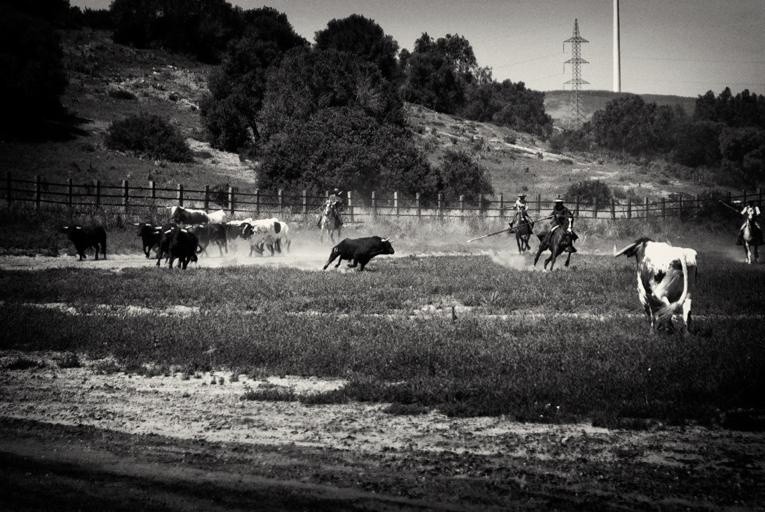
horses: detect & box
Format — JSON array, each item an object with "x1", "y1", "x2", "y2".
[
  {"x1": 533, "y1": 210, "x2": 576, "y2": 273},
  {"x1": 514, "y1": 205, "x2": 532, "y2": 254},
  {"x1": 320, "y1": 198, "x2": 341, "y2": 244},
  {"x1": 742, "y1": 204, "x2": 761, "y2": 264}
]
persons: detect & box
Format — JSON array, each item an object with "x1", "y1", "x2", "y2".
[
  {"x1": 539, "y1": 199, "x2": 578, "y2": 249},
  {"x1": 317, "y1": 189, "x2": 344, "y2": 228},
  {"x1": 509, "y1": 193, "x2": 534, "y2": 234},
  {"x1": 736, "y1": 199, "x2": 765, "y2": 244}
]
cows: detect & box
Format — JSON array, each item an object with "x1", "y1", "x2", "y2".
[
  {"x1": 64, "y1": 224, "x2": 107, "y2": 260},
  {"x1": 324, "y1": 236, "x2": 395, "y2": 271},
  {"x1": 614, "y1": 237, "x2": 697, "y2": 338},
  {"x1": 131, "y1": 217, "x2": 290, "y2": 270},
  {"x1": 166, "y1": 206, "x2": 208, "y2": 224}
]
